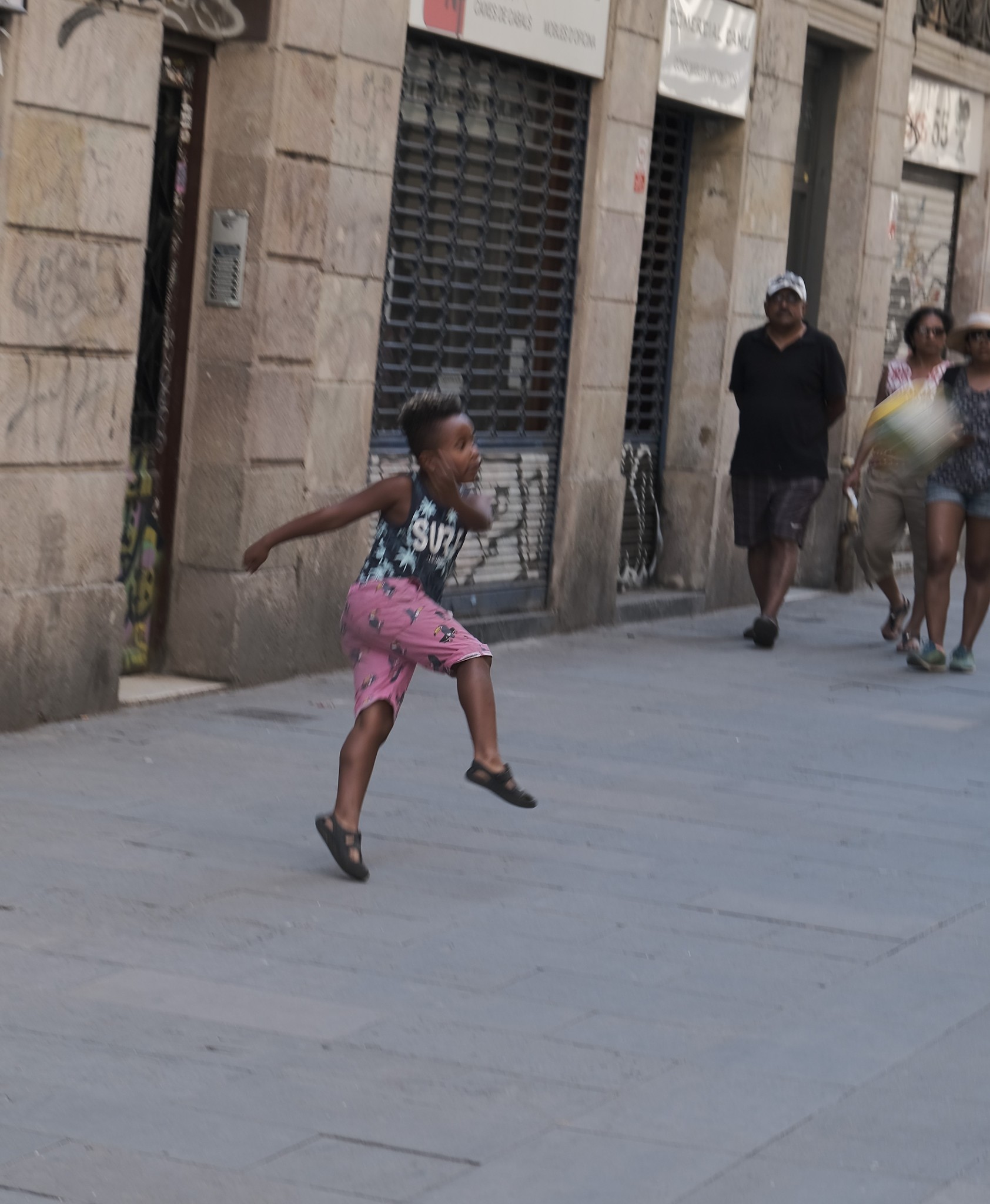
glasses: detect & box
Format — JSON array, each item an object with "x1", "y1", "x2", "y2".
[
  {"x1": 771, "y1": 294, "x2": 800, "y2": 303},
  {"x1": 918, "y1": 328, "x2": 946, "y2": 338},
  {"x1": 971, "y1": 329, "x2": 990, "y2": 339}
]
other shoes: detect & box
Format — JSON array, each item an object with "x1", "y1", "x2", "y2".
[{"x1": 743, "y1": 616, "x2": 779, "y2": 646}]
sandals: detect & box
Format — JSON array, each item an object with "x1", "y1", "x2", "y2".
[
  {"x1": 896, "y1": 630, "x2": 921, "y2": 653},
  {"x1": 466, "y1": 759, "x2": 537, "y2": 808},
  {"x1": 316, "y1": 813, "x2": 369, "y2": 878},
  {"x1": 882, "y1": 593, "x2": 911, "y2": 639}
]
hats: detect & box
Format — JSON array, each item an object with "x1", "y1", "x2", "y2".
[
  {"x1": 767, "y1": 271, "x2": 807, "y2": 303},
  {"x1": 945, "y1": 312, "x2": 990, "y2": 353}
]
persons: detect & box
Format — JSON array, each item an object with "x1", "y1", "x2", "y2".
[
  {"x1": 907, "y1": 310, "x2": 990, "y2": 673},
  {"x1": 728, "y1": 271, "x2": 848, "y2": 648},
  {"x1": 841, "y1": 305, "x2": 958, "y2": 654},
  {"x1": 241, "y1": 391, "x2": 538, "y2": 879}
]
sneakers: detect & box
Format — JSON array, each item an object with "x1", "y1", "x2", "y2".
[
  {"x1": 949, "y1": 646, "x2": 975, "y2": 670},
  {"x1": 908, "y1": 643, "x2": 948, "y2": 669}
]
